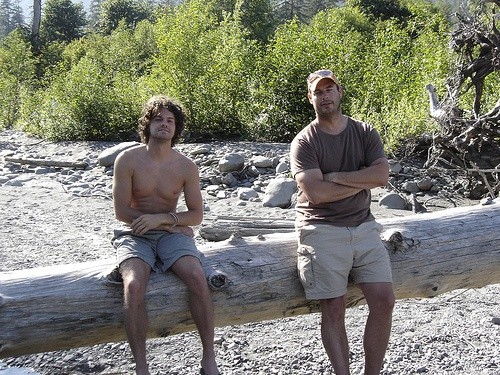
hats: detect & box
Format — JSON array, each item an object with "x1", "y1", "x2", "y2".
[{"x1": 307, "y1": 69, "x2": 341, "y2": 92}]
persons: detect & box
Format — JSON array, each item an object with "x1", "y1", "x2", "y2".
[
  {"x1": 110, "y1": 96, "x2": 222, "y2": 375},
  {"x1": 290, "y1": 70, "x2": 395, "y2": 375}
]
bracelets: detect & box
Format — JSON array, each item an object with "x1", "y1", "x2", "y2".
[{"x1": 169, "y1": 212, "x2": 179, "y2": 227}]
